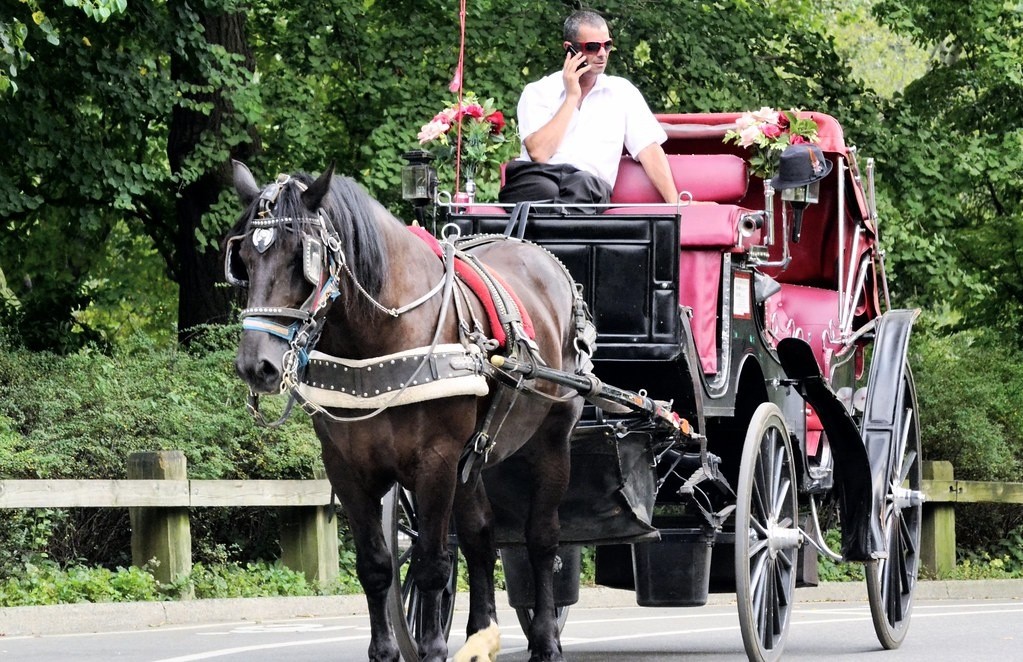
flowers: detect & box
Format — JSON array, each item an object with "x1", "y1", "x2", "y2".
[
  {"x1": 721, "y1": 106, "x2": 820, "y2": 181},
  {"x1": 416, "y1": 91, "x2": 508, "y2": 181}
]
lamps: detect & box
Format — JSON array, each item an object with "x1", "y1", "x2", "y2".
[{"x1": 401, "y1": 149, "x2": 437, "y2": 233}]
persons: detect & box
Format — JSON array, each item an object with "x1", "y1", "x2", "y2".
[{"x1": 499, "y1": 9, "x2": 687, "y2": 214}]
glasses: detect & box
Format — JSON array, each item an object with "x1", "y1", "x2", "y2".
[{"x1": 572, "y1": 38, "x2": 613, "y2": 54}]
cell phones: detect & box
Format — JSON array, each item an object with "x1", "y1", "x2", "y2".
[{"x1": 564, "y1": 45, "x2": 588, "y2": 69}]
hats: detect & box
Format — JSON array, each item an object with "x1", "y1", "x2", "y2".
[{"x1": 770, "y1": 143, "x2": 833, "y2": 190}]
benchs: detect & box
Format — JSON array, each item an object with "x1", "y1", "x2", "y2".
[
  {"x1": 756, "y1": 280, "x2": 851, "y2": 454},
  {"x1": 494, "y1": 154, "x2": 763, "y2": 376}
]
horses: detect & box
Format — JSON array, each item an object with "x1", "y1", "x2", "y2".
[{"x1": 219, "y1": 159, "x2": 587, "y2": 662}]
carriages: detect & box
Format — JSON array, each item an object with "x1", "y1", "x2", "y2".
[{"x1": 215, "y1": 103, "x2": 923, "y2": 661}]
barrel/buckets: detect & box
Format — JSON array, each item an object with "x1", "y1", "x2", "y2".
[
  {"x1": 629, "y1": 470, "x2": 719, "y2": 609},
  {"x1": 498, "y1": 542, "x2": 584, "y2": 610}
]
